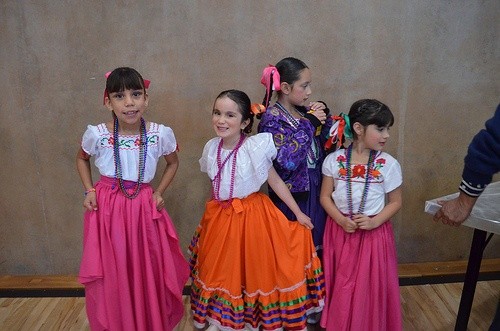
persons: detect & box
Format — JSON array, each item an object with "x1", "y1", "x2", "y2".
[
  {"x1": 320, "y1": 98, "x2": 402, "y2": 331},
  {"x1": 433, "y1": 104, "x2": 500, "y2": 228},
  {"x1": 187, "y1": 90, "x2": 325, "y2": 331},
  {"x1": 257, "y1": 57, "x2": 333, "y2": 254},
  {"x1": 76, "y1": 68, "x2": 189, "y2": 331}
]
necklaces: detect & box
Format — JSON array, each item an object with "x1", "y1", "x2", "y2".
[
  {"x1": 275, "y1": 101, "x2": 319, "y2": 165},
  {"x1": 210, "y1": 133, "x2": 246, "y2": 208},
  {"x1": 345, "y1": 142, "x2": 377, "y2": 220},
  {"x1": 111, "y1": 117, "x2": 148, "y2": 199}
]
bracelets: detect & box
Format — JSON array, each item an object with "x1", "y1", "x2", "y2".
[{"x1": 85, "y1": 189, "x2": 96, "y2": 195}]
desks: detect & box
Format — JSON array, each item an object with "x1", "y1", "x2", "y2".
[{"x1": 424, "y1": 182, "x2": 500, "y2": 331}]
